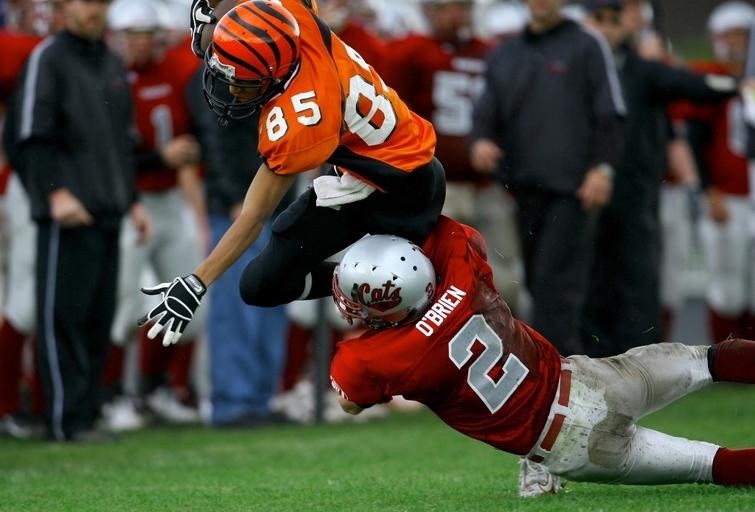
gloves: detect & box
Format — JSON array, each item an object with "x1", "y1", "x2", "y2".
[{"x1": 137, "y1": 273, "x2": 206, "y2": 347}]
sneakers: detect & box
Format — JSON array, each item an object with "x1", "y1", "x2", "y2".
[
  {"x1": 269, "y1": 383, "x2": 421, "y2": 426},
  {"x1": 190, "y1": 0, "x2": 215, "y2": 57},
  {"x1": 148, "y1": 389, "x2": 194, "y2": 423},
  {"x1": 101, "y1": 396, "x2": 141, "y2": 431}
]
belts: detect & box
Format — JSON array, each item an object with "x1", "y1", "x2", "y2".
[{"x1": 528, "y1": 355, "x2": 571, "y2": 463}]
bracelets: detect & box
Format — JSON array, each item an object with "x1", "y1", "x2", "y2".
[{"x1": 599, "y1": 165, "x2": 616, "y2": 178}]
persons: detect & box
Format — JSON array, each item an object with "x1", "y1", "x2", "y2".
[
  {"x1": 138, "y1": 3, "x2": 446, "y2": 346},
  {"x1": 0, "y1": 2, "x2": 754, "y2": 439},
  {"x1": 327, "y1": 215, "x2": 754, "y2": 493}
]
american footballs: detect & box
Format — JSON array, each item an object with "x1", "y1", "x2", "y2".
[{"x1": 200, "y1": 0, "x2": 245, "y2": 52}]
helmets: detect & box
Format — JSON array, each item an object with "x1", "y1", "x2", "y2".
[
  {"x1": 331, "y1": 232, "x2": 436, "y2": 332},
  {"x1": 201, "y1": 2, "x2": 301, "y2": 129}
]
